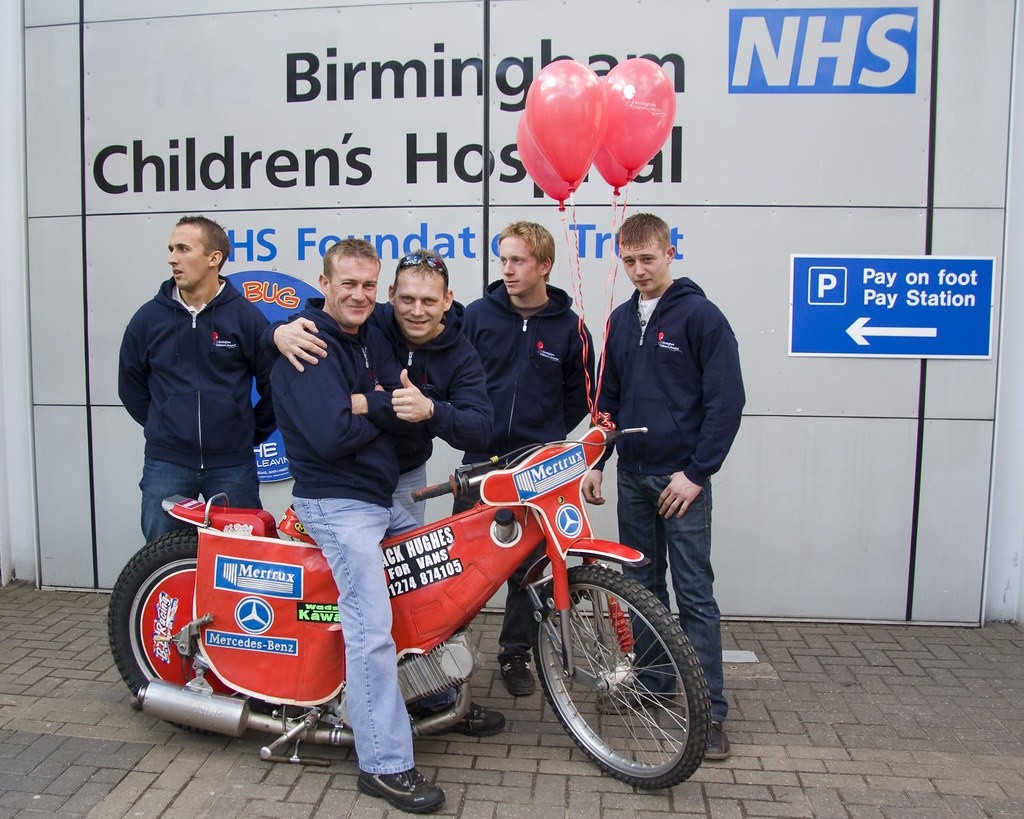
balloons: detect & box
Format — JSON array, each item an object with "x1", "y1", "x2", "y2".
[{"x1": 516, "y1": 58, "x2": 676, "y2": 212}]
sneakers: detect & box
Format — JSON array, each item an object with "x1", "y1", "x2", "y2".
[
  {"x1": 498, "y1": 650, "x2": 535, "y2": 695},
  {"x1": 704, "y1": 721, "x2": 730, "y2": 759},
  {"x1": 597, "y1": 686, "x2": 676, "y2": 714},
  {"x1": 418, "y1": 700, "x2": 506, "y2": 737},
  {"x1": 357, "y1": 767, "x2": 446, "y2": 813}
]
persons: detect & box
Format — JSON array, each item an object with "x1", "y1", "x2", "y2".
[
  {"x1": 119, "y1": 216, "x2": 278, "y2": 544},
  {"x1": 582, "y1": 213, "x2": 746, "y2": 758},
  {"x1": 259, "y1": 241, "x2": 506, "y2": 814},
  {"x1": 451, "y1": 221, "x2": 595, "y2": 696}
]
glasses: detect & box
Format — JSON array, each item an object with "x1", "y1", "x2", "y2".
[{"x1": 395, "y1": 254, "x2": 448, "y2": 294}]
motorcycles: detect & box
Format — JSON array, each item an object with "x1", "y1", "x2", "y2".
[{"x1": 107, "y1": 415, "x2": 713, "y2": 791}]
